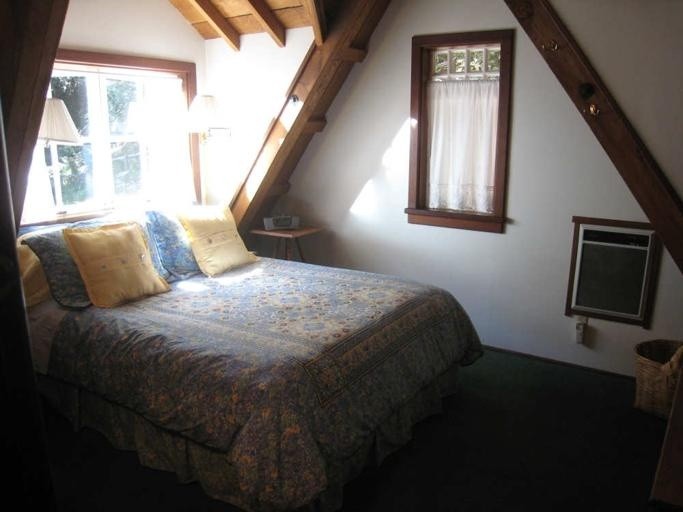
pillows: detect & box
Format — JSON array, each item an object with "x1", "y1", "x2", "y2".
[{"x1": 16, "y1": 206, "x2": 257, "y2": 311}]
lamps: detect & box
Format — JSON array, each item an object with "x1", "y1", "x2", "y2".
[
  {"x1": 38, "y1": 98, "x2": 82, "y2": 149},
  {"x1": 186, "y1": 96, "x2": 230, "y2": 142}
]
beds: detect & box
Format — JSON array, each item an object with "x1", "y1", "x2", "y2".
[{"x1": 25, "y1": 215, "x2": 485, "y2": 512}]
